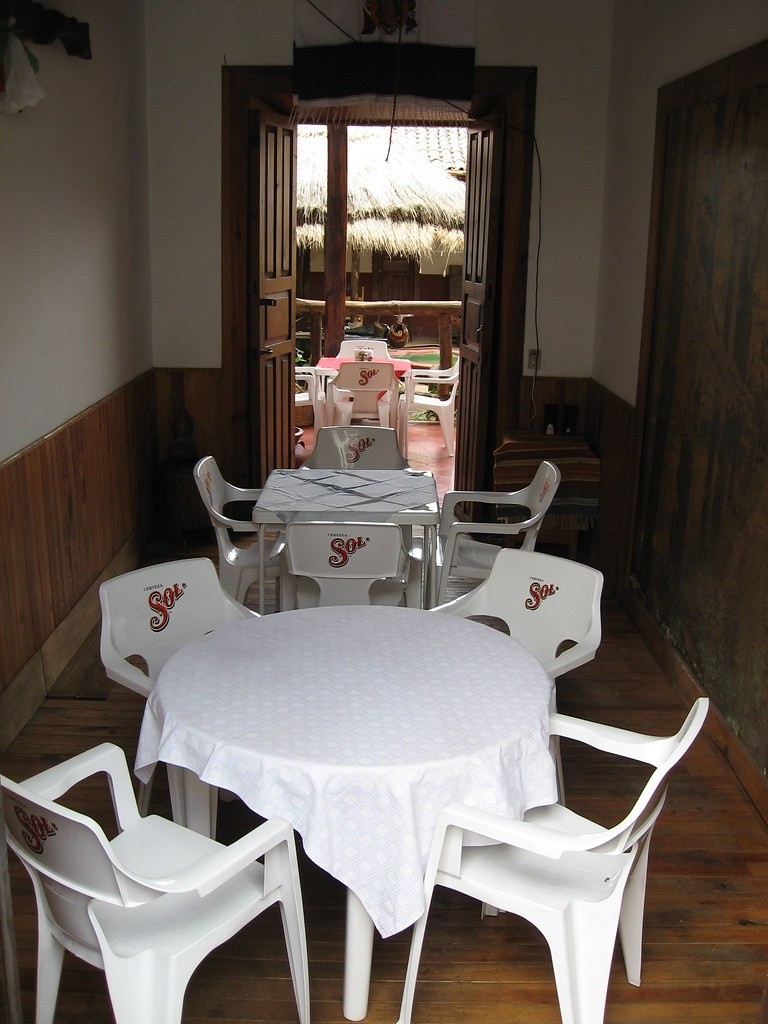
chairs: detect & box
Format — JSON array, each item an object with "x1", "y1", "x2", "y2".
[
  {"x1": 439, "y1": 458, "x2": 562, "y2": 603},
  {"x1": 300, "y1": 426, "x2": 411, "y2": 550},
  {"x1": 324, "y1": 362, "x2": 405, "y2": 444},
  {"x1": 395, "y1": 698, "x2": 713, "y2": 1024},
  {"x1": 337, "y1": 340, "x2": 392, "y2": 358},
  {"x1": 0, "y1": 740, "x2": 311, "y2": 1022},
  {"x1": 295, "y1": 366, "x2": 328, "y2": 434},
  {"x1": 397, "y1": 357, "x2": 461, "y2": 460},
  {"x1": 280, "y1": 520, "x2": 426, "y2": 611},
  {"x1": 99, "y1": 557, "x2": 260, "y2": 817},
  {"x1": 421, "y1": 547, "x2": 605, "y2": 683},
  {"x1": 192, "y1": 454, "x2": 296, "y2": 612}
]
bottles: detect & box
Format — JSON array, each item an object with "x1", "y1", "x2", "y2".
[{"x1": 355, "y1": 346, "x2": 374, "y2": 364}]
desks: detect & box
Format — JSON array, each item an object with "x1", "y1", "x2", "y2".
[
  {"x1": 136, "y1": 601, "x2": 559, "y2": 1020},
  {"x1": 493, "y1": 431, "x2": 602, "y2": 560},
  {"x1": 313, "y1": 357, "x2": 412, "y2": 459},
  {"x1": 251, "y1": 467, "x2": 441, "y2": 616}
]
